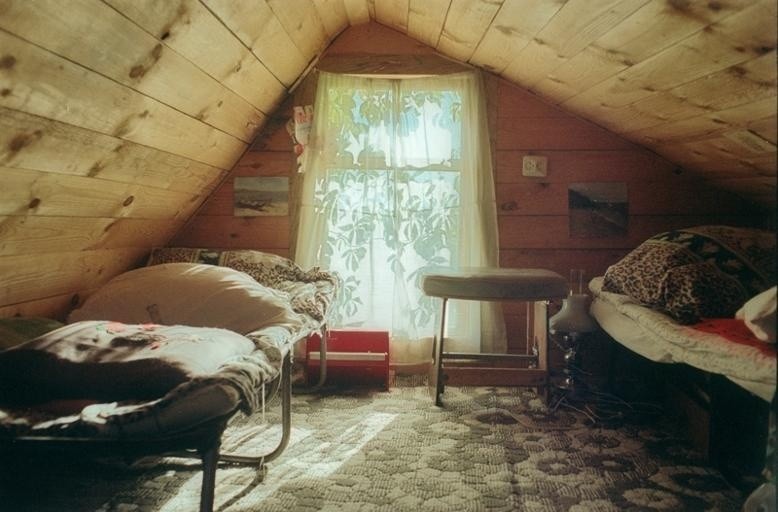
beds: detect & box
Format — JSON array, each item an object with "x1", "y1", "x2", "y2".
[
  {"x1": 590, "y1": 224, "x2": 778, "y2": 478},
  {"x1": 0, "y1": 247, "x2": 341, "y2": 512}
]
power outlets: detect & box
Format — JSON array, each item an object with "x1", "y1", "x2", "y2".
[{"x1": 523, "y1": 155, "x2": 548, "y2": 177}]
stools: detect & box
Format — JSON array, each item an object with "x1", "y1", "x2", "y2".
[{"x1": 420, "y1": 269, "x2": 570, "y2": 407}]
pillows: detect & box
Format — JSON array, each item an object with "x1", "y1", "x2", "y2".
[
  {"x1": 0, "y1": 319, "x2": 257, "y2": 402},
  {"x1": 67, "y1": 263, "x2": 289, "y2": 336},
  {"x1": 735, "y1": 283, "x2": 778, "y2": 349}
]
